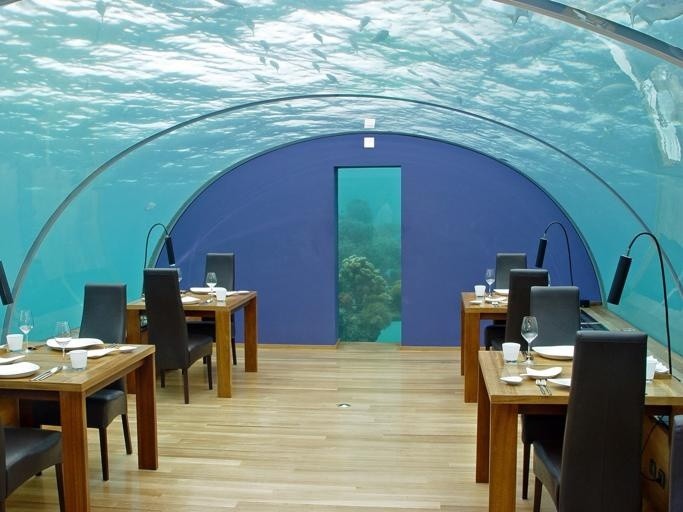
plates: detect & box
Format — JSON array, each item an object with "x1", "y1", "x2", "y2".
[
  {"x1": 499, "y1": 345, "x2": 574, "y2": 387},
  {"x1": 46, "y1": 337, "x2": 103, "y2": 351},
  {"x1": 0, "y1": 356, "x2": 25, "y2": 364},
  {"x1": 469, "y1": 289, "x2": 509, "y2": 304},
  {"x1": 0, "y1": 362, "x2": 39, "y2": 379},
  {"x1": 182, "y1": 287, "x2": 250, "y2": 304}
]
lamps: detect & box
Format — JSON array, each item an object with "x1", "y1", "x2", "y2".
[
  {"x1": 143, "y1": 223, "x2": 175, "y2": 268},
  {"x1": 0, "y1": 261, "x2": 14, "y2": 305},
  {"x1": 607, "y1": 232, "x2": 672, "y2": 375},
  {"x1": 536, "y1": 222, "x2": 574, "y2": 286}
]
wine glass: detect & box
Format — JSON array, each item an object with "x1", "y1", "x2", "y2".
[
  {"x1": 485, "y1": 269, "x2": 495, "y2": 298},
  {"x1": 521, "y1": 315, "x2": 539, "y2": 363},
  {"x1": 206, "y1": 272, "x2": 217, "y2": 301},
  {"x1": 176, "y1": 268, "x2": 182, "y2": 282},
  {"x1": 55, "y1": 321, "x2": 71, "y2": 366},
  {"x1": 19, "y1": 310, "x2": 34, "y2": 353}
]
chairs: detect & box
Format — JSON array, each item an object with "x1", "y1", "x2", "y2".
[
  {"x1": 0, "y1": 427, "x2": 65, "y2": 512},
  {"x1": 522, "y1": 286, "x2": 580, "y2": 499},
  {"x1": 485, "y1": 253, "x2": 527, "y2": 350},
  {"x1": 32, "y1": 285, "x2": 132, "y2": 482},
  {"x1": 533, "y1": 330, "x2": 647, "y2": 512},
  {"x1": 202, "y1": 253, "x2": 237, "y2": 365},
  {"x1": 144, "y1": 268, "x2": 212, "y2": 404},
  {"x1": 491, "y1": 269, "x2": 548, "y2": 350}
]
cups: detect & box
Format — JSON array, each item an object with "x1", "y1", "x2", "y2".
[
  {"x1": 475, "y1": 285, "x2": 485, "y2": 296},
  {"x1": 6, "y1": 334, "x2": 23, "y2": 351},
  {"x1": 70, "y1": 351, "x2": 87, "y2": 369},
  {"x1": 502, "y1": 342, "x2": 519, "y2": 363},
  {"x1": 646, "y1": 363, "x2": 656, "y2": 382}
]
